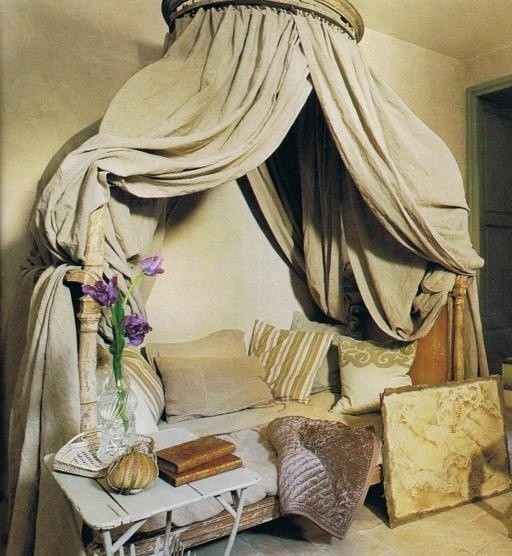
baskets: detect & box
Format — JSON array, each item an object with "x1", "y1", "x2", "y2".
[{"x1": 53, "y1": 429, "x2": 157, "y2": 478}]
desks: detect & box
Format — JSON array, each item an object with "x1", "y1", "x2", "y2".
[{"x1": 42, "y1": 423, "x2": 261, "y2": 556}]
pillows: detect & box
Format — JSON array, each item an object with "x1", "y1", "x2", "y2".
[
  {"x1": 289, "y1": 309, "x2": 366, "y2": 401},
  {"x1": 249, "y1": 321, "x2": 334, "y2": 406},
  {"x1": 96, "y1": 345, "x2": 165, "y2": 443},
  {"x1": 155, "y1": 355, "x2": 278, "y2": 423},
  {"x1": 327, "y1": 334, "x2": 418, "y2": 416},
  {"x1": 146, "y1": 329, "x2": 247, "y2": 382}
]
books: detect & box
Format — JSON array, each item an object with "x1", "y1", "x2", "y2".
[
  {"x1": 154, "y1": 433, "x2": 236, "y2": 473},
  {"x1": 158, "y1": 452, "x2": 243, "y2": 488}
]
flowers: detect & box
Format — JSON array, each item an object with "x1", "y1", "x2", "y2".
[{"x1": 82, "y1": 248, "x2": 165, "y2": 435}]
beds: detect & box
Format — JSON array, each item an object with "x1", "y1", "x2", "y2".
[{"x1": 2, "y1": 259, "x2": 485, "y2": 556}]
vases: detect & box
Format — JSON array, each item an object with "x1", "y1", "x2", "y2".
[{"x1": 95, "y1": 369, "x2": 139, "y2": 467}]
